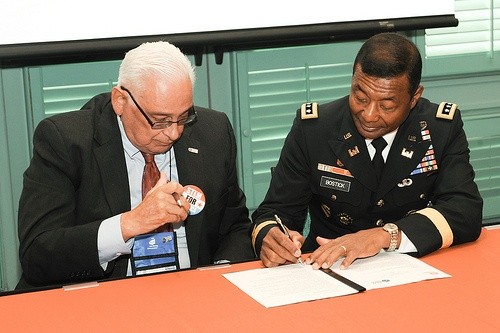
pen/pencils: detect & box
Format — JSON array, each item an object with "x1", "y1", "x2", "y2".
[
  {"x1": 275, "y1": 214, "x2": 304, "y2": 266},
  {"x1": 166, "y1": 180, "x2": 182, "y2": 207}
]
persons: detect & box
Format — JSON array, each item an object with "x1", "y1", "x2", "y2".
[
  {"x1": 12, "y1": 40, "x2": 255, "y2": 291},
  {"x1": 251, "y1": 32, "x2": 484, "y2": 270}
]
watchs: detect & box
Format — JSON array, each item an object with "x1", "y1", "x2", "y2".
[{"x1": 382, "y1": 223, "x2": 400, "y2": 252}]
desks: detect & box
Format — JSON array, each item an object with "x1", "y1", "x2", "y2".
[{"x1": 0, "y1": 223, "x2": 500, "y2": 333}]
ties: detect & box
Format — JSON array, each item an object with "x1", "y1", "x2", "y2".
[
  {"x1": 140, "y1": 151, "x2": 174, "y2": 233},
  {"x1": 371, "y1": 136, "x2": 388, "y2": 184}
]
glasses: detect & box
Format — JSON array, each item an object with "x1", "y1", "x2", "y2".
[{"x1": 120, "y1": 85, "x2": 198, "y2": 130}]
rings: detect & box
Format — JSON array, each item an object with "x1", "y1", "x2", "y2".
[
  {"x1": 176, "y1": 214, "x2": 182, "y2": 224},
  {"x1": 341, "y1": 245, "x2": 348, "y2": 253}
]
